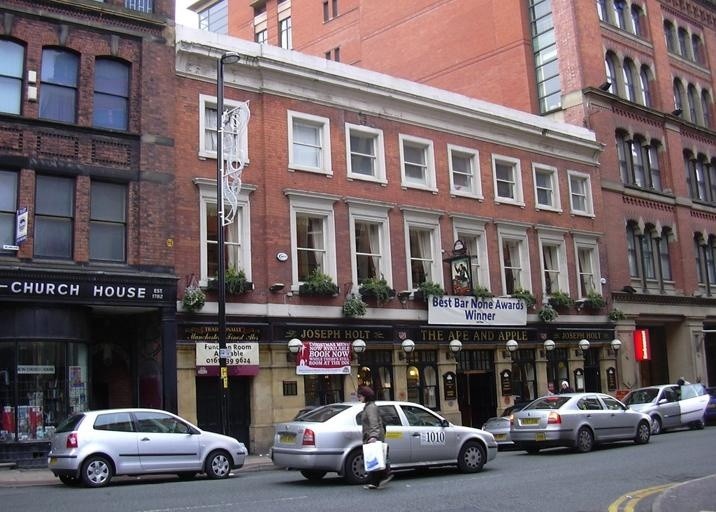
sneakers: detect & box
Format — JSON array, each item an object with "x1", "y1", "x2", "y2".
[{"x1": 364, "y1": 475, "x2": 389, "y2": 489}]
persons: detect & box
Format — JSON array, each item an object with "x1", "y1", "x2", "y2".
[
  {"x1": 544, "y1": 383, "x2": 557, "y2": 394},
  {"x1": 453, "y1": 264, "x2": 469, "y2": 285},
  {"x1": 559, "y1": 379, "x2": 574, "y2": 395},
  {"x1": 357, "y1": 384, "x2": 393, "y2": 489}
]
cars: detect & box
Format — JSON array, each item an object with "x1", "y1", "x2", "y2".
[
  {"x1": 270, "y1": 400, "x2": 499, "y2": 485},
  {"x1": 481, "y1": 399, "x2": 555, "y2": 452},
  {"x1": 45, "y1": 408, "x2": 250, "y2": 488},
  {"x1": 703, "y1": 386, "x2": 716, "y2": 425},
  {"x1": 620, "y1": 381, "x2": 712, "y2": 436},
  {"x1": 509, "y1": 392, "x2": 655, "y2": 455}
]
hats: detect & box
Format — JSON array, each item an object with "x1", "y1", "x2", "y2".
[{"x1": 359, "y1": 386, "x2": 374, "y2": 396}]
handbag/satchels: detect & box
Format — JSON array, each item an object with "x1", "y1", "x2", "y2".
[{"x1": 363, "y1": 441, "x2": 387, "y2": 471}]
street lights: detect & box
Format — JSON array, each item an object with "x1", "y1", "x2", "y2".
[{"x1": 216, "y1": 53, "x2": 239, "y2": 437}]
